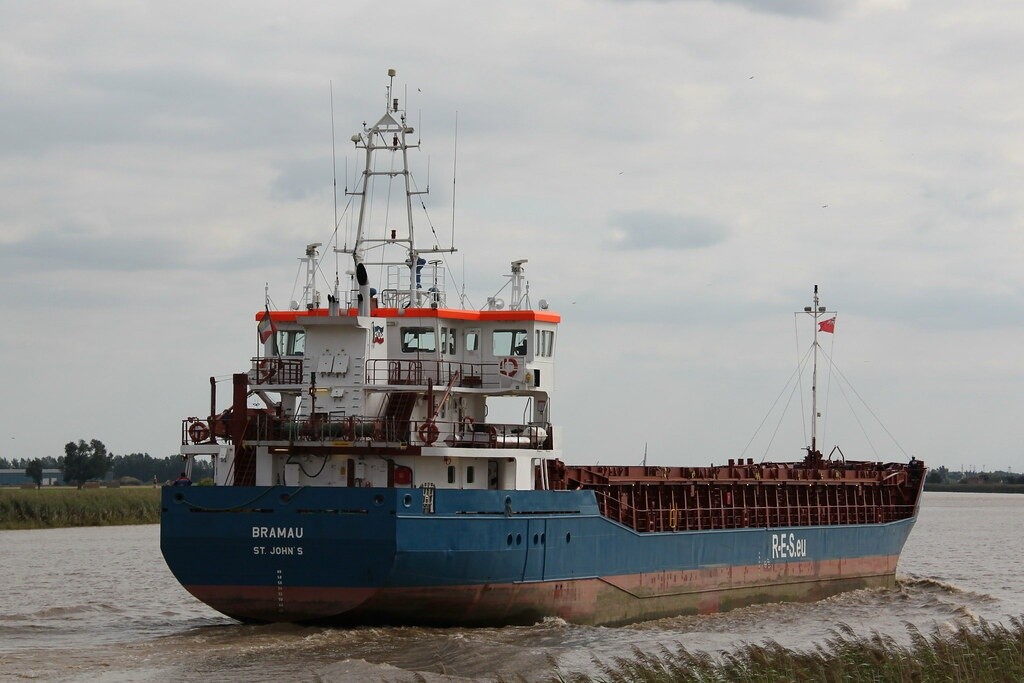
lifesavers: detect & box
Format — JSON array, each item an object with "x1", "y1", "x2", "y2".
[
  {"x1": 419, "y1": 423, "x2": 439, "y2": 444},
  {"x1": 189, "y1": 422, "x2": 209, "y2": 442},
  {"x1": 376, "y1": 420, "x2": 381, "y2": 438},
  {"x1": 344, "y1": 418, "x2": 351, "y2": 435},
  {"x1": 260, "y1": 359, "x2": 276, "y2": 378},
  {"x1": 500, "y1": 358, "x2": 518, "y2": 377},
  {"x1": 395, "y1": 467, "x2": 411, "y2": 485}
]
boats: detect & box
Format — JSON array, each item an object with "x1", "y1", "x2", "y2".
[{"x1": 155, "y1": 68, "x2": 929, "y2": 620}]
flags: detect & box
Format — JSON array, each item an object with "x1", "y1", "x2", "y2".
[{"x1": 818, "y1": 317, "x2": 835, "y2": 334}]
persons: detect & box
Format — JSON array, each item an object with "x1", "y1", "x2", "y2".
[{"x1": 513, "y1": 339, "x2": 528, "y2": 355}]
